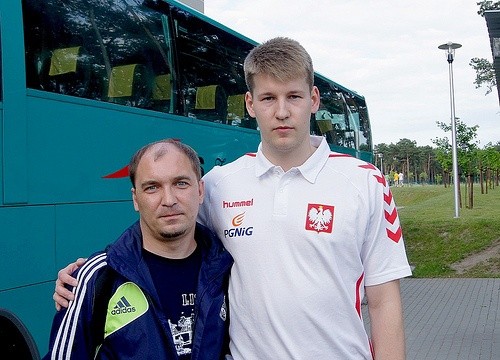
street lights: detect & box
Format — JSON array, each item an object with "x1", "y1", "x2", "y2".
[{"x1": 437, "y1": 42, "x2": 464, "y2": 219}]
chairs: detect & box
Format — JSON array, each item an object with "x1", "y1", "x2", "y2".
[
  {"x1": 314, "y1": 120, "x2": 344, "y2": 144},
  {"x1": 174, "y1": 85, "x2": 228, "y2": 124},
  {"x1": 33, "y1": 44, "x2": 94, "y2": 99},
  {"x1": 149, "y1": 74, "x2": 172, "y2": 112},
  {"x1": 225, "y1": 94, "x2": 258, "y2": 131},
  {"x1": 91, "y1": 64, "x2": 152, "y2": 106}
]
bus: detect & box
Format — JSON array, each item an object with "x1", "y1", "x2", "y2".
[{"x1": 0, "y1": 0, "x2": 380, "y2": 360}]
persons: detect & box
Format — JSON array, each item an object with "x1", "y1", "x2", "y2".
[
  {"x1": 385, "y1": 170, "x2": 403, "y2": 186},
  {"x1": 42, "y1": 139, "x2": 234, "y2": 360},
  {"x1": 52, "y1": 36, "x2": 413, "y2": 360}
]
